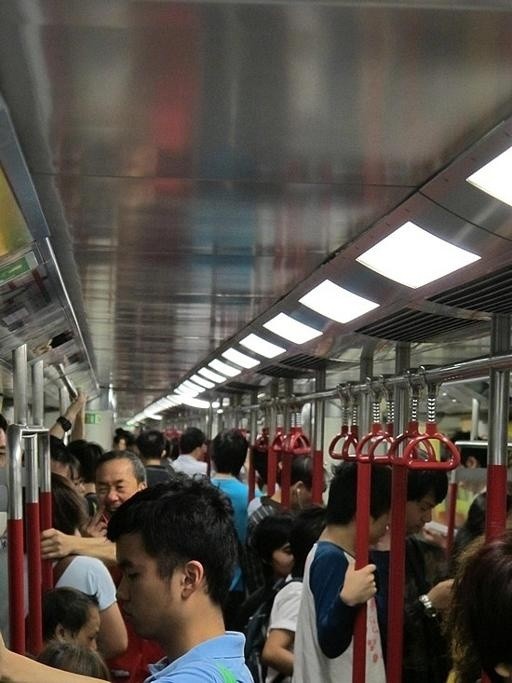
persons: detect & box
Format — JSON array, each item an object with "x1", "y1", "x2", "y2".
[
  {"x1": 210, "y1": 427, "x2": 511, "y2": 682},
  {"x1": 0, "y1": 386, "x2": 253, "y2": 683}
]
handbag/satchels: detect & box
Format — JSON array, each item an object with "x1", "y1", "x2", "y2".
[{"x1": 243, "y1": 578, "x2": 302, "y2": 683}]
